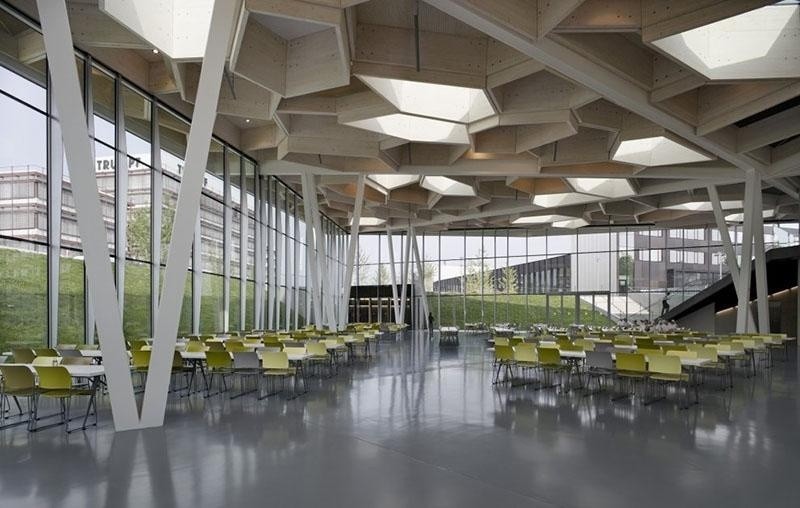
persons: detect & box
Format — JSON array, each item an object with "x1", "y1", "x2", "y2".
[
  {"x1": 615, "y1": 317, "x2": 678, "y2": 332},
  {"x1": 661, "y1": 292, "x2": 671, "y2": 317}
]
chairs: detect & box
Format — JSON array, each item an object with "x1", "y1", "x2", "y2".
[
  {"x1": 437, "y1": 322, "x2": 487, "y2": 352},
  {"x1": 1, "y1": 345, "x2": 105, "y2": 432},
  {"x1": 128, "y1": 323, "x2": 409, "y2": 401},
  {"x1": 486, "y1": 324, "x2": 798, "y2": 409}
]
